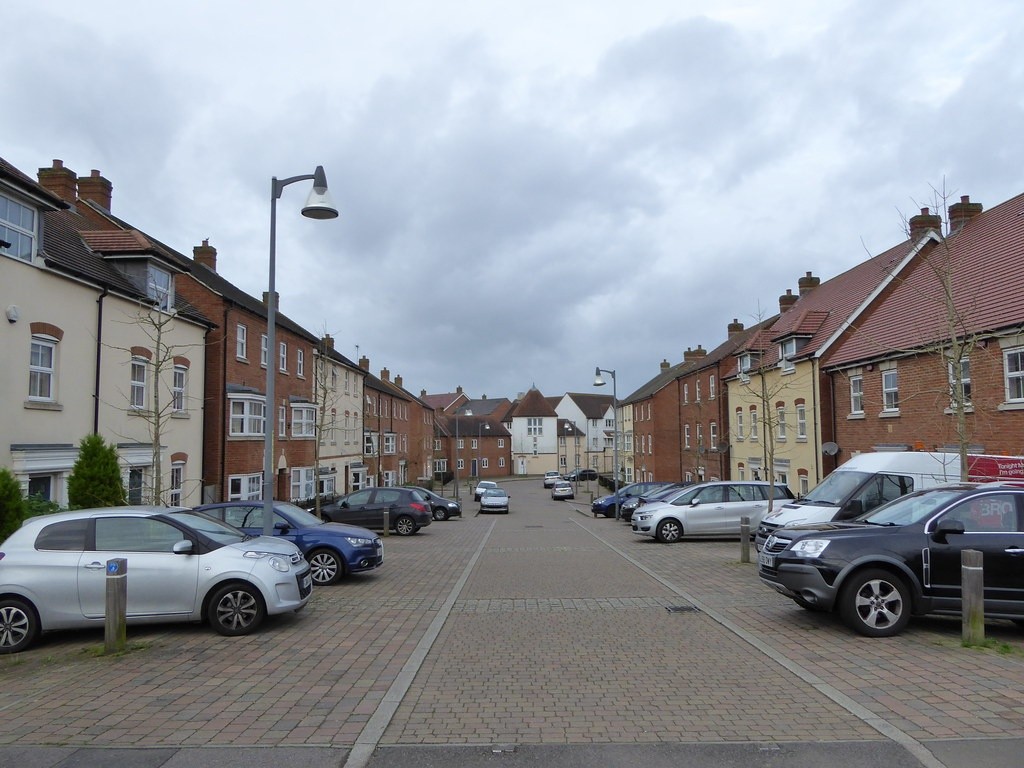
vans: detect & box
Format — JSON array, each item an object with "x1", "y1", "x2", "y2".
[{"x1": 757, "y1": 452, "x2": 1023, "y2": 557}]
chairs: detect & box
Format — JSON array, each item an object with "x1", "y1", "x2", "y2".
[{"x1": 1001, "y1": 511, "x2": 1016, "y2": 532}]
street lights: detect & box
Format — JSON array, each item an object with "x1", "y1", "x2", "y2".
[
  {"x1": 594, "y1": 365, "x2": 620, "y2": 520},
  {"x1": 559, "y1": 417, "x2": 594, "y2": 495},
  {"x1": 263, "y1": 166, "x2": 340, "y2": 537},
  {"x1": 477, "y1": 421, "x2": 493, "y2": 496},
  {"x1": 454, "y1": 402, "x2": 473, "y2": 501}
]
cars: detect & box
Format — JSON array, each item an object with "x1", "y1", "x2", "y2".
[
  {"x1": 544, "y1": 471, "x2": 573, "y2": 502},
  {"x1": 563, "y1": 469, "x2": 596, "y2": 482},
  {"x1": 474, "y1": 480, "x2": 514, "y2": 515},
  {"x1": 0, "y1": 504, "x2": 315, "y2": 655},
  {"x1": 583, "y1": 482, "x2": 795, "y2": 543},
  {"x1": 409, "y1": 487, "x2": 463, "y2": 522},
  {"x1": 192, "y1": 499, "x2": 386, "y2": 583},
  {"x1": 304, "y1": 488, "x2": 433, "y2": 535}
]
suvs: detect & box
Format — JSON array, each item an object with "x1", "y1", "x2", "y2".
[{"x1": 757, "y1": 486, "x2": 1024, "y2": 636}]
